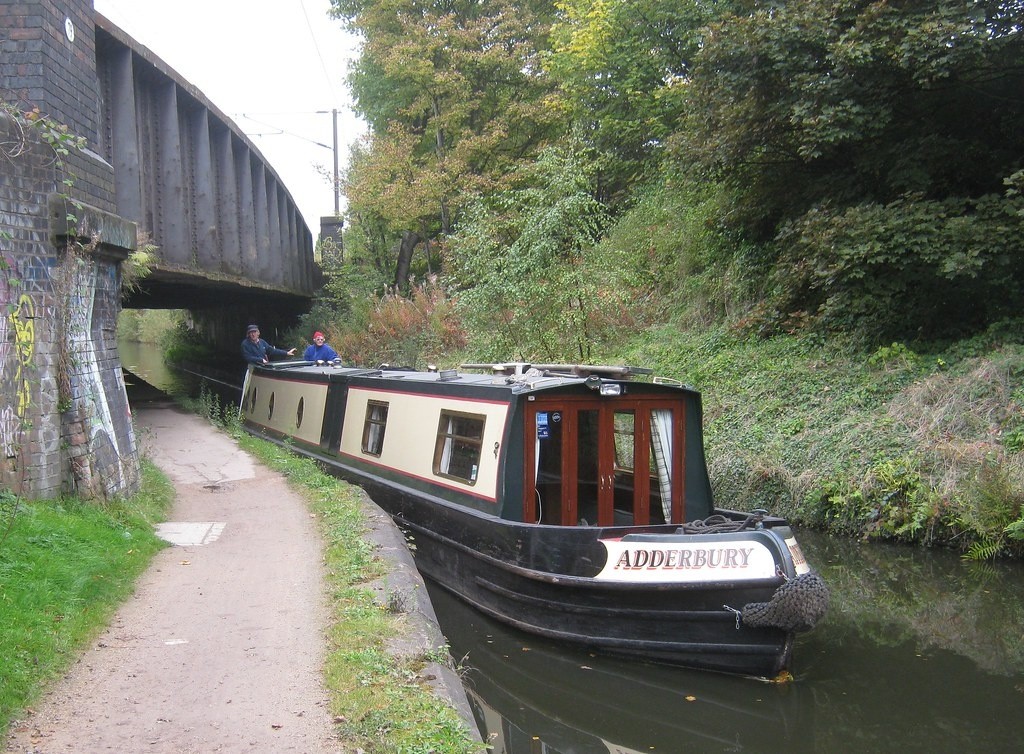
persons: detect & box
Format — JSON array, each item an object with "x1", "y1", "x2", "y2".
[
  {"x1": 240, "y1": 324, "x2": 298, "y2": 363},
  {"x1": 304, "y1": 331, "x2": 342, "y2": 365}
]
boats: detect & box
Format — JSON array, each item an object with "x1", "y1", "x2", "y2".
[{"x1": 232, "y1": 356, "x2": 832, "y2": 683}]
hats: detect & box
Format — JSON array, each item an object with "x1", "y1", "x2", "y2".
[
  {"x1": 313, "y1": 331, "x2": 324, "y2": 339},
  {"x1": 246, "y1": 325, "x2": 258, "y2": 332}
]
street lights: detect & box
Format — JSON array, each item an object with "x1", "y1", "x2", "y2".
[{"x1": 315, "y1": 107, "x2": 341, "y2": 217}]
